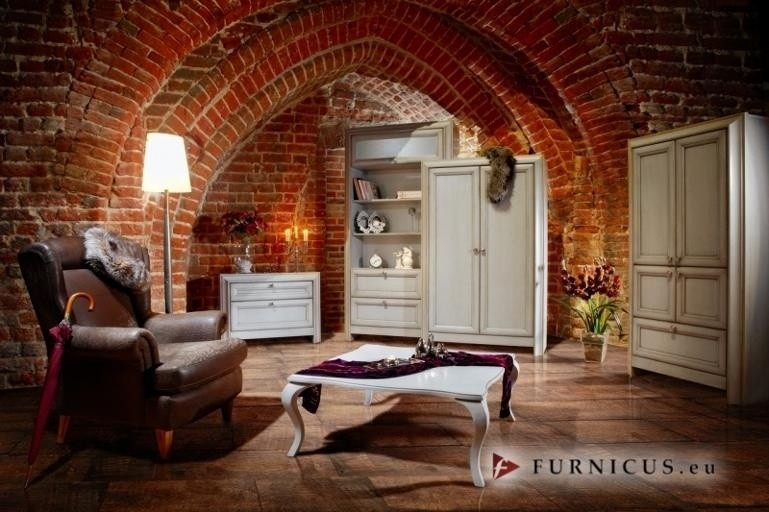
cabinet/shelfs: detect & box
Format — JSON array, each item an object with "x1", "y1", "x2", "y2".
[
  {"x1": 420, "y1": 154, "x2": 549, "y2": 357},
  {"x1": 343, "y1": 119, "x2": 454, "y2": 342},
  {"x1": 627, "y1": 111, "x2": 768, "y2": 405},
  {"x1": 219, "y1": 271, "x2": 321, "y2": 343}
]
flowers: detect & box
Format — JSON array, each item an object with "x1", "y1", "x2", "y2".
[
  {"x1": 550, "y1": 256, "x2": 629, "y2": 339},
  {"x1": 219, "y1": 209, "x2": 269, "y2": 248}
]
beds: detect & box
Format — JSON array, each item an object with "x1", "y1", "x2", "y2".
[{"x1": 279, "y1": 344, "x2": 520, "y2": 486}]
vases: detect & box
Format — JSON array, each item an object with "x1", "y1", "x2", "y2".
[
  {"x1": 581, "y1": 330, "x2": 610, "y2": 364},
  {"x1": 232, "y1": 238, "x2": 256, "y2": 272}
]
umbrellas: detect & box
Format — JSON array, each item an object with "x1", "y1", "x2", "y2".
[{"x1": 24, "y1": 292, "x2": 94, "y2": 489}]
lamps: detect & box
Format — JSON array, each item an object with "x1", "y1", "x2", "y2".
[{"x1": 140, "y1": 132, "x2": 194, "y2": 313}]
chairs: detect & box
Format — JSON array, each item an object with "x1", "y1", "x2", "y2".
[{"x1": 15, "y1": 235, "x2": 249, "y2": 460}]
